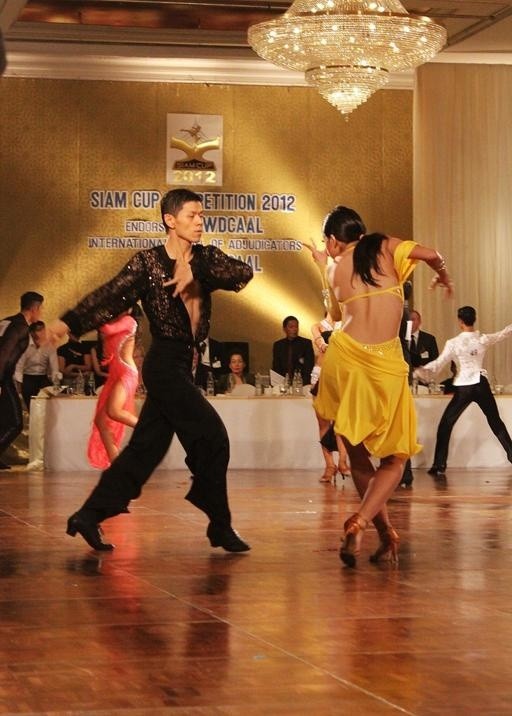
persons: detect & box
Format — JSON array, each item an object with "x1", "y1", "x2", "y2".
[
  {"x1": 399, "y1": 291, "x2": 512, "y2": 483},
  {"x1": 301, "y1": 204, "x2": 455, "y2": 566},
  {"x1": 192, "y1": 313, "x2": 350, "y2": 483},
  {"x1": 0, "y1": 290, "x2": 143, "y2": 471},
  {"x1": 35, "y1": 186, "x2": 255, "y2": 552}
]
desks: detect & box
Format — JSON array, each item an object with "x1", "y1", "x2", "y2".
[{"x1": 29, "y1": 393, "x2": 511, "y2": 474}]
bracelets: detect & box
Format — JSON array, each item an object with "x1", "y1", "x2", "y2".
[
  {"x1": 436, "y1": 263, "x2": 447, "y2": 273},
  {"x1": 430, "y1": 259, "x2": 445, "y2": 270}
]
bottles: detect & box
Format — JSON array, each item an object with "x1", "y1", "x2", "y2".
[
  {"x1": 205, "y1": 370, "x2": 305, "y2": 399},
  {"x1": 51, "y1": 370, "x2": 96, "y2": 397},
  {"x1": 428, "y1": 379, "x2": 437, "y2": 394},
  {"x1": 412, "y1": 378, "x2": 418, "y2": 395}
]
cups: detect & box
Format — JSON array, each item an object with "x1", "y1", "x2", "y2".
[
  {"x1": 494, "y1": 384, "x2": 505, "y2": 395},
  {"x1": 436, "y1": 385, "x2": 446, "y2": 395}
]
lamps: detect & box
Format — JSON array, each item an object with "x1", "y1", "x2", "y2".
[{"x1": 246, "y1": 0, "x2": 448, "y2": 125}]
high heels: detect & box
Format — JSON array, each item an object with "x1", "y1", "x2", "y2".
[
  {"x1": 318, "y1": 463, "x2": 339, "y2": 484},
  {"x1": 338, "y1": 513, "x2": 368, "y2": 570},
  {"x1": 338, "y1": 460, "x2": 351, "y2": 480},
  {"x1": 369, "y1": 528, "x2": 403, "y2": 566}
]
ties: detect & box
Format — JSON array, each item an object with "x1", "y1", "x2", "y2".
[{"x1": 410, "y1": 334, "x2": 416, "y2": 353}]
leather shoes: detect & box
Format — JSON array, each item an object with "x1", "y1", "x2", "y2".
[
  {"x1": 65, "y1": 511, "x2": 117, "y2": 553},
  {"x1": 427, "y1": 462, "x2": 447, "y2": 475},
  {"x1": 205, "y1": 520, "x2": 253, "y2": 553},
  {"x1": 399, "y1": 470, "x2": 414, "y2": 485}
]
